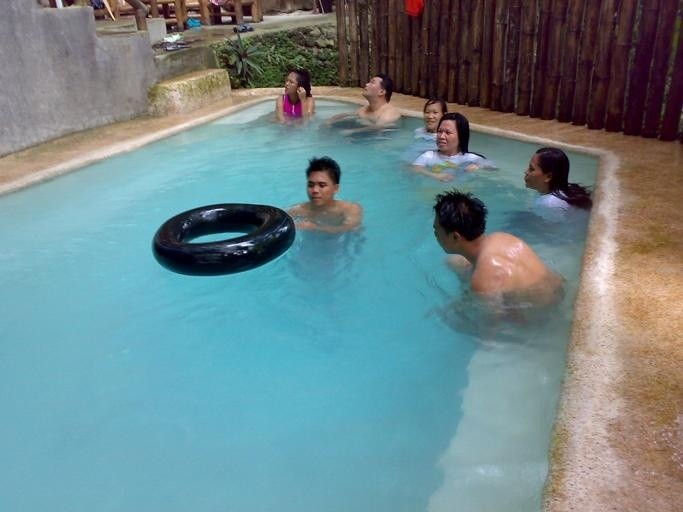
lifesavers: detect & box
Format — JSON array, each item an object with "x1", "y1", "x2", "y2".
[{"x1": 151, "y1": 202, "x2": 294, "y2": 274}]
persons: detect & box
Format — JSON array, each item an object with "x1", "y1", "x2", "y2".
[
  {"x1": 324, "y1": 74, "x2": 401, "y2": 137},
  {"x1": 285, "y1": 154, "x2": 363, "y2": 234},
  {"x1": 409, "y1": 113, "x2": 498, "y2": 181},
  {"x1": 414, "y1": 97, "x2": 446, "y2": 140},
  {"x1": 276, "y1": 69, "x2": 314, "y2": 122},
  {"x1": 524, "y1": 147, "x2": 592, "y2": 222},
  {"x1": 432, "y1": 186, "x2": 567, "y2": 352}
]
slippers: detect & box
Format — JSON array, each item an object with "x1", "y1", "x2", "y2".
[
  {"x1": 153, "y1": 35, "x2": 204, "y2": 51},
  {"x1": 244, "y1": 24, "x2": 252, "y2": 31},
  {"x1": 234, "y1": 26, "x2": 247, "y2": 32}
]
